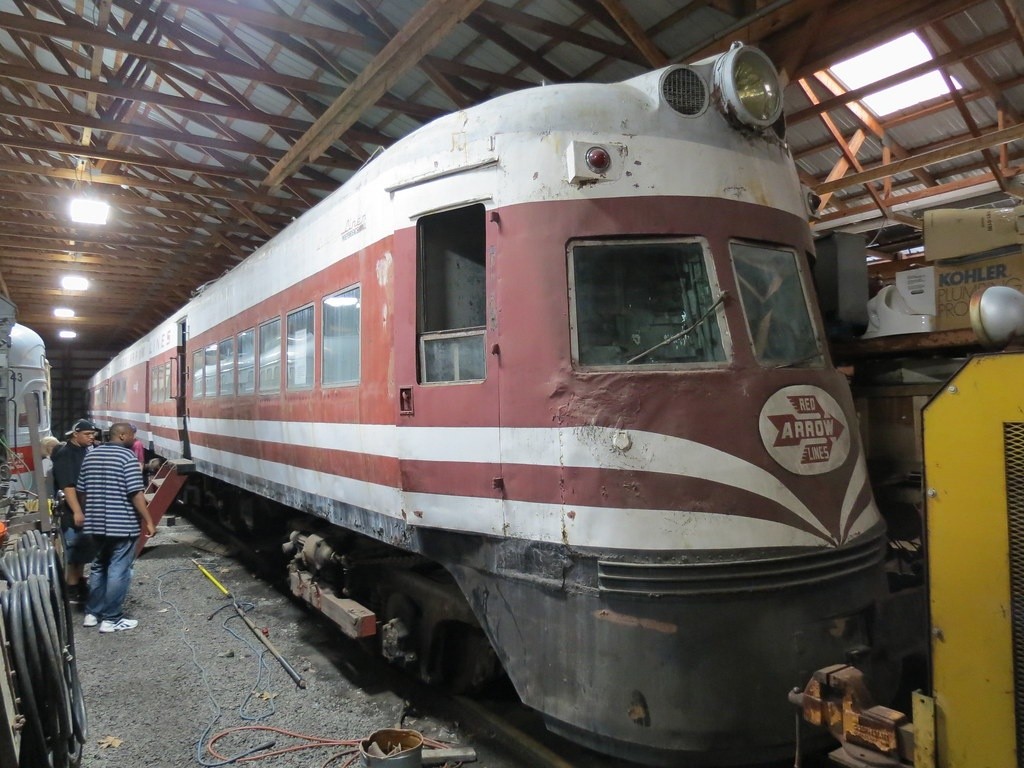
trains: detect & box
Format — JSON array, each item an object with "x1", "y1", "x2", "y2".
[
  {"x1": 84, "y1": 38, "x2": 901, "y2": 768},
  {"x1": 7, "y1": 323, "x2": 54, "y2": 503}
]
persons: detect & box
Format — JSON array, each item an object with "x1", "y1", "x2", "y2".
[
  {"x1": 39, "y1": 437, "x2": 61, "y2": 499},
  {"x1": 53, "y1": 419, "x2": 97, "y2": 600},
  {"x1": 129, "y1": 425, "x2": 144, "y2": 472},
  {"x1": 77, "y1": 423, "x2": 156, "y2": 633}
]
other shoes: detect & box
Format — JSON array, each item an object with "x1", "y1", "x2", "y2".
[{"x1": 64, "y1": 576, "x2": 91, "y2": 604}]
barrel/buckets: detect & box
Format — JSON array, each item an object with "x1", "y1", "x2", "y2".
[{"x1": 359, "y1": 728, "x2": 424, "y2": 768}]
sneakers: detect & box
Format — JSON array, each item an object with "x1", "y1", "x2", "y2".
[
  {"x1": 99, "y1": 618, "x2": 138, "y2": 633},
  {"x1": 83, "y1": 614, "x2": 98, "y2": 626}
]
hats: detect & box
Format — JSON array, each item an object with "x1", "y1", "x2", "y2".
[{"x1": 65, "y1": 419, "x2": 96, "y2": 436}]
